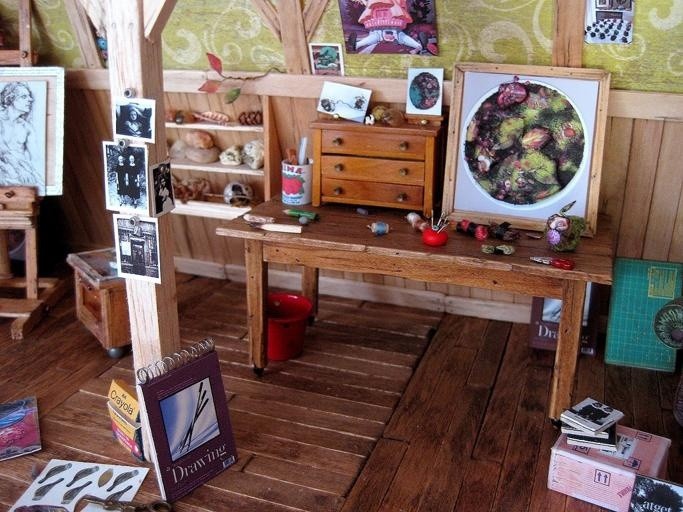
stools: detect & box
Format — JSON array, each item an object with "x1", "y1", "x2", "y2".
[{"x1": 65, "y1": 243, "x2": 131, "y2": 360}]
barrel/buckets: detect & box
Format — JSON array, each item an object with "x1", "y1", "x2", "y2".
[{"x1": 264, "y1": 290, "x2": 314, "y2": 363}]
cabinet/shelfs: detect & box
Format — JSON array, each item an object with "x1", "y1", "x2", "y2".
[
  {"x1": 309, "y1": 115, "x2": 441, "y2": 218},
  {"x1": 164, "y1": 93, "x2": 283, "y2": 224}
]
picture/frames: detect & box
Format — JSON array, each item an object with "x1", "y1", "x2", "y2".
[{"x1": 438, "y1": 60, "x2": 611, "y2": 242}]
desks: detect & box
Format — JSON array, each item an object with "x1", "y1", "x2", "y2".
[{"x1": 211, "y1": 192, "x2": 614, "y2": 431}]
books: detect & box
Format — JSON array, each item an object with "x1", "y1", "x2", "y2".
[{"x1": 559, "y1": 397, "x2": 624, "y2": 452}]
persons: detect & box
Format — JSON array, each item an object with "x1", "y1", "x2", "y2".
[
  {"x1": 137, "y1": 248, "x2": 142, "y2": 261},
  {"x1": 0, "y1": 81, "x2": 42, "y2": 185},
  {"x1": 158, "y1": 178, "x2": 173, "y2": 211},
  {"x1": 115, "y1": 155, "x2": 129, "y2": 205},
  {"x1": 120, "y1": 105, "x2": 147, "y2": 136},
  {"x1": 125, "y1": 154, "x2": 140, "y2": 204}
]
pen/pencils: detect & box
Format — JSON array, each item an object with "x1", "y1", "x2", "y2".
[
  {"x1": 286, "y1": 137, "x2": 307, "y2": 165},
  {"x1": 242, "y1": 209, "x2": 321, "y2": 234}
]
